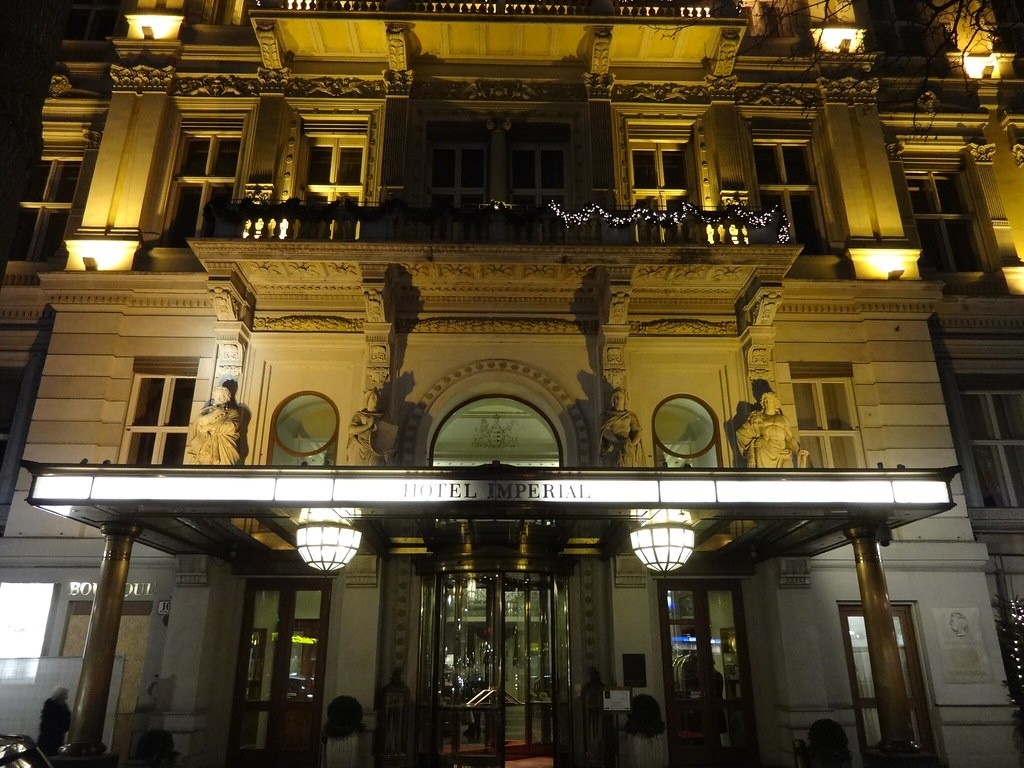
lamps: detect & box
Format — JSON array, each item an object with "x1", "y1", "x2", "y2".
[
  {"x1": 296, "y1": 523, "x2": 362, "y2": 572},
  {"x1": 630, "y1": 523, "x2": 695, "y2": 574}
]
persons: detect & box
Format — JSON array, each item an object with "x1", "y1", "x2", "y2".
[
  {"x1": 711, "y1": 659, "x2": 727, "y2": 753},
  {"x1": 735, "y1": 391, "x2": 809, "y2": 469},
  {"x1": 595, "y1": 388, "x2": 645, "y2": 466},
  {"x1": 184, "y1": 379, "x2": 243, "y2": 466},
  {"x1": 37, "y1": 686, "x2": 71, "y2": 758},
  {"x1": 346, "y1": 388, "x2": 397, "y2": 466}
]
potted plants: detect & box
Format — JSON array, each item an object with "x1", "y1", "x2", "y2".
[
  {"x1": 619, "y1": 695, "x2": 667, "y2": 768},
  {"x1": 323, "y1": 697, "x2": 368, "y2": 767},
  {"x1": 134, "y1": 730, "x2": 180, "y2": 768},
  {"x1": 801, "y1": 719, "x2": 853, "y2": 768}
]
